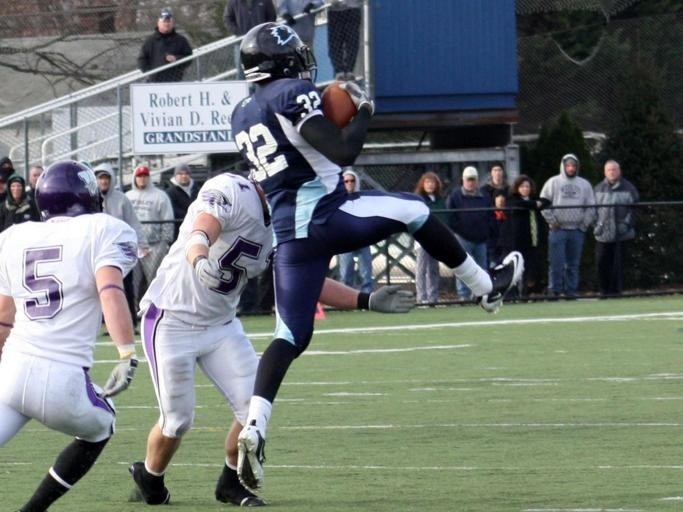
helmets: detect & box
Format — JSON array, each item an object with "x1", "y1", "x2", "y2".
[
  {"x1": 35, "y1": 160, "x2": 104, "y2": 219},
  {"x1": 239, "y1": 22, "x2": 317, "y2": 83}
]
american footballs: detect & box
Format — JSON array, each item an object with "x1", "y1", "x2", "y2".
[{"x1": 321, "y1": 82, "x2": 354, "y2": 129}]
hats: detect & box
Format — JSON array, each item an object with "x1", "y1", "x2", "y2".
[
  {"x1": 136, "y1": 167, "x2": 149, "y2": 176},
  {"x1": 159, "y1": 6, "x2": 175, "y2": 21},
  {"x1": 175, "y1": 163, "x2": 191, "y2": 176},
  {"x1": 463, "y1": 166, "x2": 479, "y2": 180},
  {"x1": 565, "y1": 157, "x2": 576, "y2": 164},
  {"x1": 97, "y1": 172, "x2": 109, "y2": 177}
]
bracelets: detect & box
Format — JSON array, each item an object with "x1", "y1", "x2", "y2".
[
  {"x1": 96, "y1": 285, "x2": 127, "y2": 296},
  {"x1": 114, "y1": 344, "x2": 138, "y2": 357},
  {"x1": 193, "y1": 255, "x2": 206, "y2": 269},
  {"x1": 357, "y1": 292, "x2": 371, "y2": 309},
  {"x1": 183, "y1": 229, "x2": 212, "y2": 255}
]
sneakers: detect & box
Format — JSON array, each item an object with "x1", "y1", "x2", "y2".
[
  {"x1": 215, "y1": 477, "x2": 265, "y2": 506},
  {"x1": 236, "y1": 425, "x2": 265, "y2": 491},
  {"x1": 129, "y1": 461, "x2": 170, "y2": 504},
  {"x1": 480, "y1": 251, "x2": 524, "y2": 312}
]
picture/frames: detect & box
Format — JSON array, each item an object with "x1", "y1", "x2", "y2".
[{"x1": 130, "y1": 80, "x2": 249, "y2": 154}]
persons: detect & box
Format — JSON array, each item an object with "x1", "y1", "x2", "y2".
[
  {"x1": 277, "y1": 0, "x2": 324, "y2": 64},
  {"x1": 124, "y1": 166, "x2": 175, "y2": 312},
  {"x1": 127, "y1": 168, "x2": 417, "y2": 508},
  {"x1": 0, "y1": 156, "x2": 45, "y2": 232},
  {"x1": 223, "y1": 0, "x2": 277, "y2": 80},
  {"x1": 230, "y1": 20, "x2": 526, "y2": 498},
  {"x1": 326, "y1": 0, "x2": 361, "y2": 80},
  {"x1": 164, "y1": 162, "x2": 198, "y2": 246},
  {"x1": 93, "y1": 162, "x2": 150, "y2": 336},
  {"x1": 137, "y1": 8, "x2": 193, "y2": 82},
  {"x1": 338, "y1": 169, "x2": 374, "y2": 295},
  {"x1": 0, "y1": 158, "x2": 141, "y2": 512},
  {"x1": 412, "y1": 152, "x2": 639, "y2": 308}
]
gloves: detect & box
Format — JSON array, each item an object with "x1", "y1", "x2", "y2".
[
  {"x1": 369, "y1": 286, "x2": 415, "y2": 313},
  {"x1": 102, "y1": 358, "x2": 138, "y2": 399},
  {"x1": 340, "y1": 81, "x2": 375, "y2": 114},
  {"x1": 193, "y1": 257, "x2": 220, "y2": 288}
]
